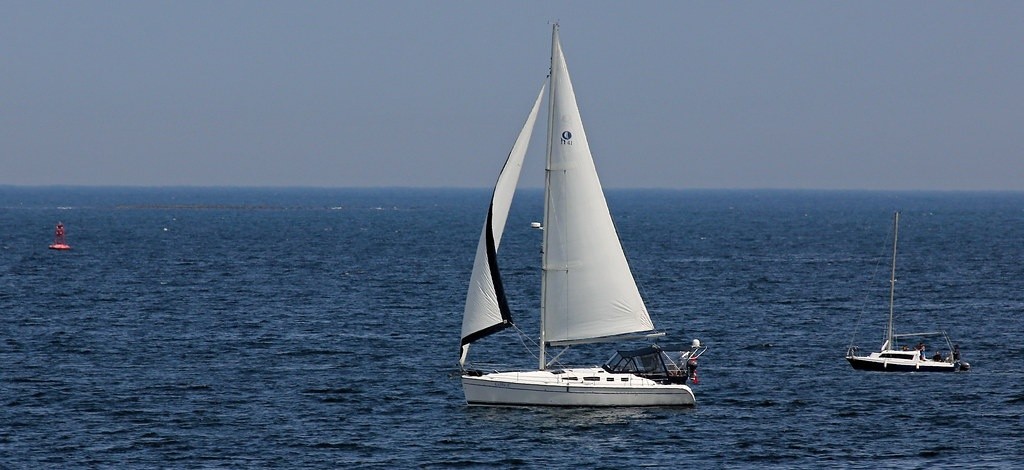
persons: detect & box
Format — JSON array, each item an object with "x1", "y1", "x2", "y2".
[
  {"x1": 933, "y1": 351, "x2": 943, "y2": 362},
  {"x1": 914, "y1": 343, "x2": 925, "y2": 359},
  {"x1": 953, "y1": 345, "x2": 961, "y2": 370}
]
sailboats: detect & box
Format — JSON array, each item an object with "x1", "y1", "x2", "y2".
[
  {"x1": 844, "y1": 211, "x2": 972, "y2": 373},
  {"x1": 458, "y1": 22, "x2": 708, "y2": 408}
]
